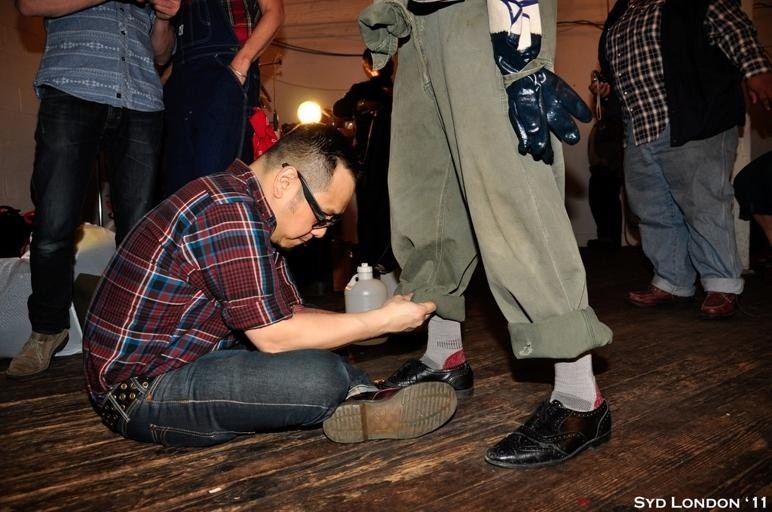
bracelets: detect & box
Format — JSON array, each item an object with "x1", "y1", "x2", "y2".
[{"x1": 229, "y1": 63, "x2": 247, "y2": 78}]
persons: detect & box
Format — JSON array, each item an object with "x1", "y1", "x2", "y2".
[
  {"x1": 733, "y1": 150, "x2": 772, "y2": 248},
  {"x1": 83, "y1": 124, "x2": 457, "y2": 448},
  {"x1": 588, "y1": 108, "x2": 655, "y2": 289},
  {"x1": 589, "y1": 0, "x2": 771, "y2": 321},
  {"x1": 333, "y1": 49, "x2": 392, "y2": 267},
  {"x1": 153, "y1": 0, "x2": 285, "y2": 211},
  {"x1": 357, "y1": 0, "x2": 615, "y2": 469},
  {"x1": 5, "y1": 0, "x2": 181, "y2": 382},
  {"x1": 0, "y1": 205, "x2": 30, "y2": 258}
]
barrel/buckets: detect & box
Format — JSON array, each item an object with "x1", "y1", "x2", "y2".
[{"x1": 344, "y1": 263, "x2": 390, "y2": 347}]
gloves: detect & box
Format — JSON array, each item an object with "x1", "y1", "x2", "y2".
[{"x1": 486, "y1": 1, "x2": 593, "y2": 165}]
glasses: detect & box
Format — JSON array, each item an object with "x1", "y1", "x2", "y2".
[{"x1": 282, "y1": 163, "x2": 344, "y2": 229}]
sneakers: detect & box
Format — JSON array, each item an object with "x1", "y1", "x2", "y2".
[
  {"x1": 624, "y1": 283, "x2": 697, "y2": 308},
  {"x1": 5, "y1": 327, "x2": 69, "y2": 380},
  {"x1": 698, "y1": 291, "x2": 739, "y2": 319}
]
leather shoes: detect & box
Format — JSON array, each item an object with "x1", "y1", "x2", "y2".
[
  {"x1": 375, "y1": 358, "x2": 474, "y2": 400},
  {"x1": 322, "y1": 381, "x2": 458, "y2": 444},
  {"x1": 484, "y1": 397, "x2": 611, "y2": 469}
]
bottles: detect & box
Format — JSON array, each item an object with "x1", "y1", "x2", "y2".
[{"x1": 343, "y1": 261, "x2": 389, "y2": 346}]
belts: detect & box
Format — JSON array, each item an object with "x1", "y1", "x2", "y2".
[{"x1": 100, "y1": 376, "x2": 153, "y2": 434}]
